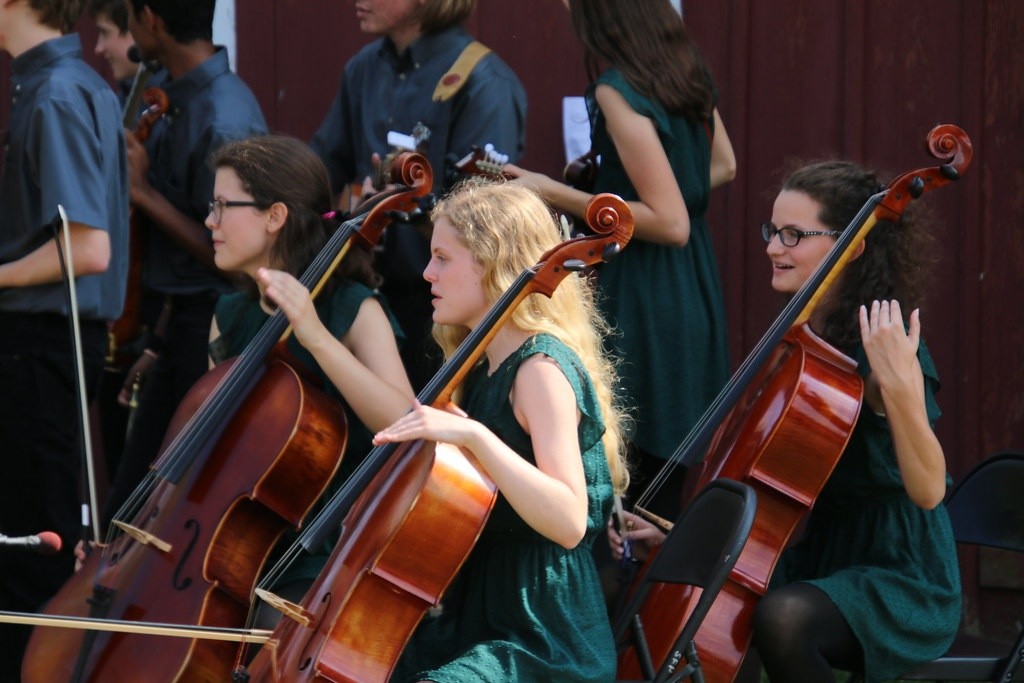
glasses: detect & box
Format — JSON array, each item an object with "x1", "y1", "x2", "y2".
[
  {"x1": 207, "y1": 199, "x2": 258, "y2": 224},
  {"x1": 759, "y1": 222, "x2": 843, "y2": 247}
]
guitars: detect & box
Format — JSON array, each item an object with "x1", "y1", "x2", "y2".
[
  {"x1": 455, "y1": 142, "x2": 602, "y2": 236},
  {"x1": 336, "y1": 123, "x2": 432, "y2": 214}
]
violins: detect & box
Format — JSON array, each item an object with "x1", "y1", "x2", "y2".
[{"x1": 99, "y1": 85, "x2": 175, "y2": 342}]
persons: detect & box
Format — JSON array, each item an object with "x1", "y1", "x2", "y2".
[
  {"x1": 606, "y1": 159, "x2": 964, "y2": 683},
  {"x1": 0, "y1": 0, "x2": 739, "y2": 683}
]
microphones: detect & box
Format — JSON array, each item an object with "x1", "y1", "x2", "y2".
[{"x1": 0, "y1": 532, "x2": 62, "y2": 554}]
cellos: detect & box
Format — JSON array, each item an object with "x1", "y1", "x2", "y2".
[
  {"x1": 229, "y1": 192, "x2": 635, "y2": 682},
  {"x1": 20, "y1": 149, "x2": 438, "y2": 682},
  {"x1": 611, "y1": 122, "x2": 977, "y2": 683}
]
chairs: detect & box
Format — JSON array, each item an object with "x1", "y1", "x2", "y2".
[
  {"x1": 614, "y1": 477, "x2": 757, "y2": 683},
  {"x1": 901, "y1": 452, "x2": 1024, "y2": 683}
]
